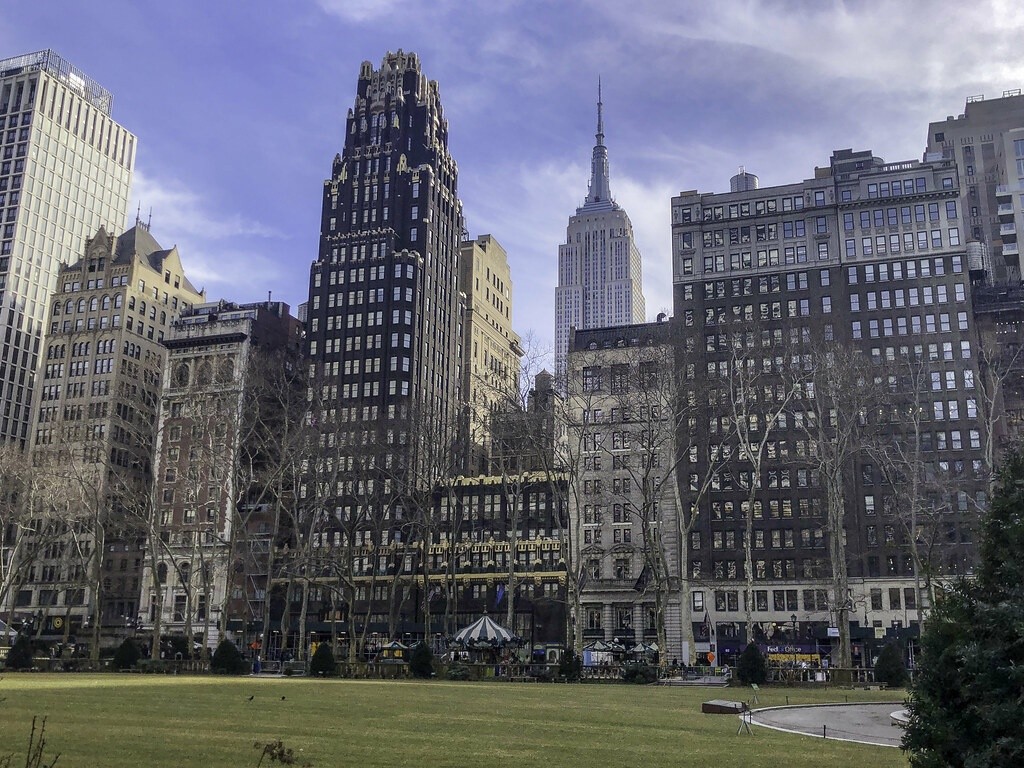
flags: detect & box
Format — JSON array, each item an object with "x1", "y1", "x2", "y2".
[
  {"x1": 702, "y1": 611, "x2": 711, "y2": 636},
  {"x1": 420, "y1": 584, "x2": 435, "y2": 611},
  {"x1": 633, "y1": 563, "x2": 652, "y2": 593},
  {"x1": 494, "y1": 582, "x2": 504, "y2": 606},
  {"x1": 576, "y1": 566, "x2": 590, "y2": 594}
]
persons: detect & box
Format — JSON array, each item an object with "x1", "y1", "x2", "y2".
[
  {"x1": 771, "y1": 658, "x2": 866, "y2": 681},
  {"x1": 600, "y1": 656, "x2": 701, "y2": 679}
]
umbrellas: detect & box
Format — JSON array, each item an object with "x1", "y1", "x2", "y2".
[
  {"x1": 382, "y1": 640, "x2": 409, "y2": 662},
  {"x1": 583, "y1": 639, "x2": 659, "y2": 666}
]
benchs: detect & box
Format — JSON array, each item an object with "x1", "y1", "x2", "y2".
[{"x1": 860, "y1": 682, "x2": 888, "y2": 690}]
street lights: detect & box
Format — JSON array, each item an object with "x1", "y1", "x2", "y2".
[
  {"x1": 791, "y1": 613, "x2": 797, "y2": 663},
  {"x1": 807, "y1": 624, "x2": 813, "y2": 667}
]
